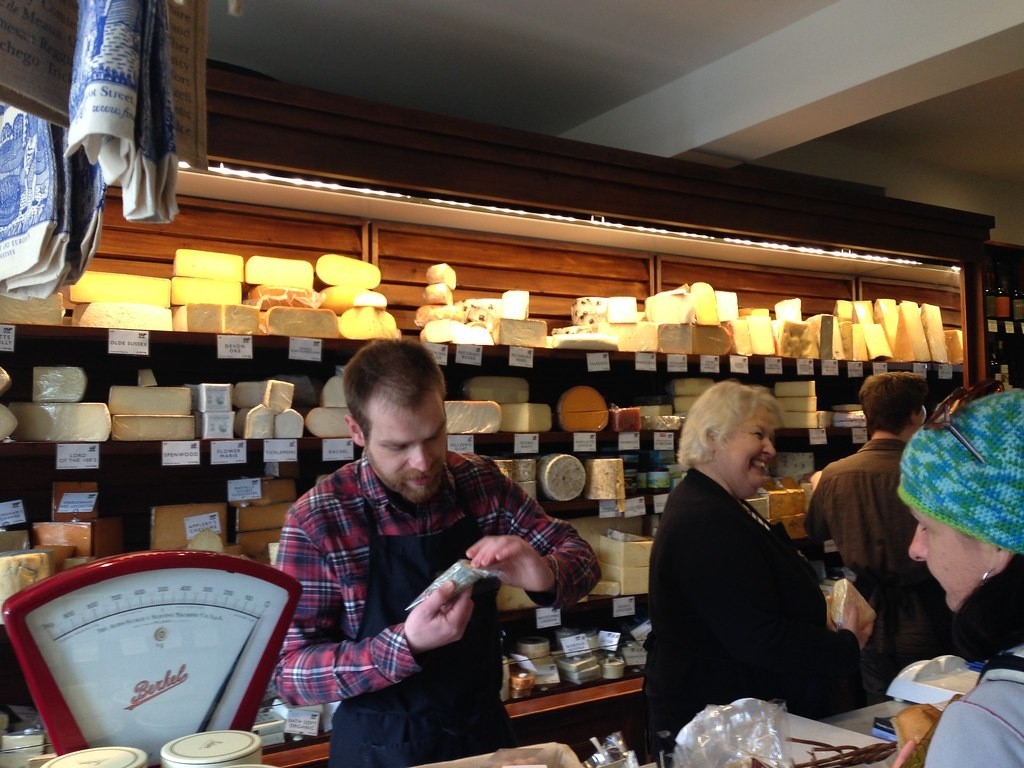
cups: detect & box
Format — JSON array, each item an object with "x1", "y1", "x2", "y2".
[
  {"x1": 159, "y1": 729, "x2": 263, "y2": 768},
  {"x1": 39, "y1": 746, "x2": 148, "y2": 768}
]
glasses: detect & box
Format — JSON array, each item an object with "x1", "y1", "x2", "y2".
[{"x1": 925, "y1": 381, "x2": 1004, "y2": 463}]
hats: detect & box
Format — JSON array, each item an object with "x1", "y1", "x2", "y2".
[{"x1": 897, "y1": 389, "x2": 1024, "y2": 553}]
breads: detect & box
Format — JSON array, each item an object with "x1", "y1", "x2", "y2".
[
  {"x1": 0, "y1": 248, "x2": 964, "y2": 627},
  {"x1": 897, "y1": 703, "x2": 943, "y2": 748}
]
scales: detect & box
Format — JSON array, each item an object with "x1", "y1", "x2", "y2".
[{"x1": 3, "y1": 549, "x2": 303, "y2": 768}]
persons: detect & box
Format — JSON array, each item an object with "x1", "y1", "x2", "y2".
[
  {"x1": 269, "y1": 336, "x2": 601, "y2": 768},
  {"x1": 638, "y1": 383, "x2": 876, "y2": 757},
  {"x1": 894, "y1": 386, "x2": 1024, "y2": 768},
  {"x1": 805, "y1": 366, "x2": 955, "y2": 706}
]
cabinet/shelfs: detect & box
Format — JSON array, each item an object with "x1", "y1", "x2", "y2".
[{"x1": 0, "y1": 69, "x2": 1024, "y2": 768}]
